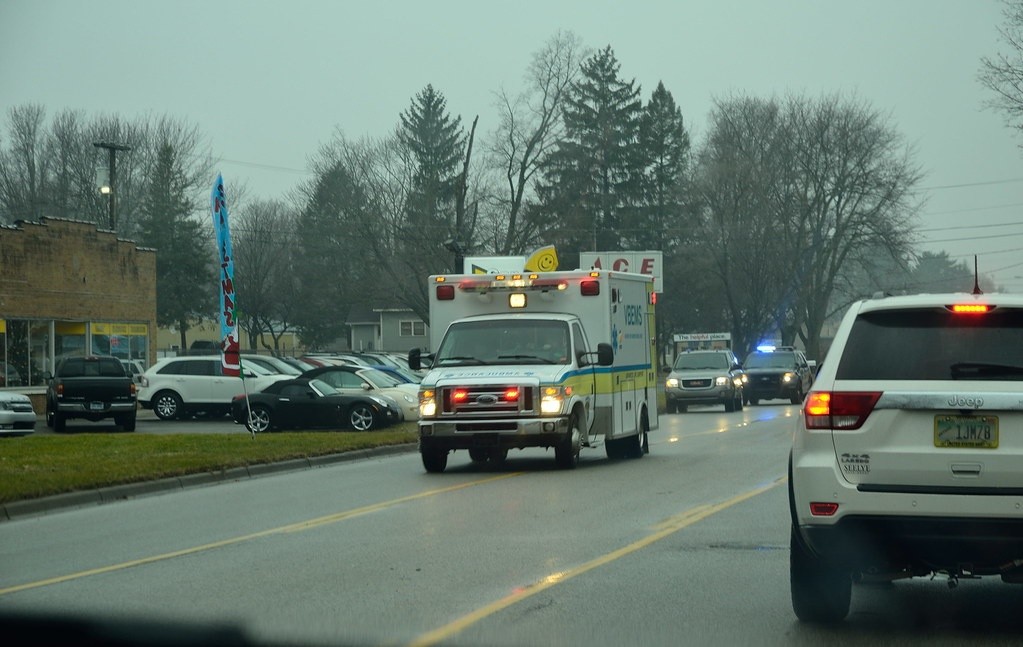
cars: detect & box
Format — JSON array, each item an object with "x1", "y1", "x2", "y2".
[
  {"x1": 122, "y1": 359, "x2": 144, "y2": 387},
  {"x1": 0, "y1": 391, "x2": 36, "y2": 436},
  {"x1": 295, "y1": 367, "x2": 420, "y2": 422},
  {"x1": 242, "y1": 352, "x2": 433, "y2": 386},
  {"x1": 0, "y1": 362, "x2": 21, "y2": 386}
]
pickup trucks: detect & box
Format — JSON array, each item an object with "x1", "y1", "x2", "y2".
[{"x1": 42, "y1": 357, "x2": 138, "y2": 433}]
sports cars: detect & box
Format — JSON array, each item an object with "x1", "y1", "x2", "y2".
[{"x1": 229, "y1": 379, "x2": 396, "y2": 432}]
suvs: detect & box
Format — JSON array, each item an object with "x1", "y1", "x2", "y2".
[
  {"x1": 663, "y1": 346, "x2": 744, "y2": 413},
  {"x1": 787, "y1": 291, "x2": 1022, "y2": 625},
  {"x1": 137, "y1": 355, "x2": 299, "y2": 421},
  {"x1": 741, "y1": 344, "x2": 812, "y2": 405}
]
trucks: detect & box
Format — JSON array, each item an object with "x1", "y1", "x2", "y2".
[{"x1": 407, "y1": 270, "x2": 658, "y2": 474}]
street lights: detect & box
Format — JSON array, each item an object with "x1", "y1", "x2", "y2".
[
  {"x1": 100, "y1": 185, "x2": 116, "y2": 230},
  {"x1": 442, "y1": 240, "x2": 465, "y2": 274}
]
